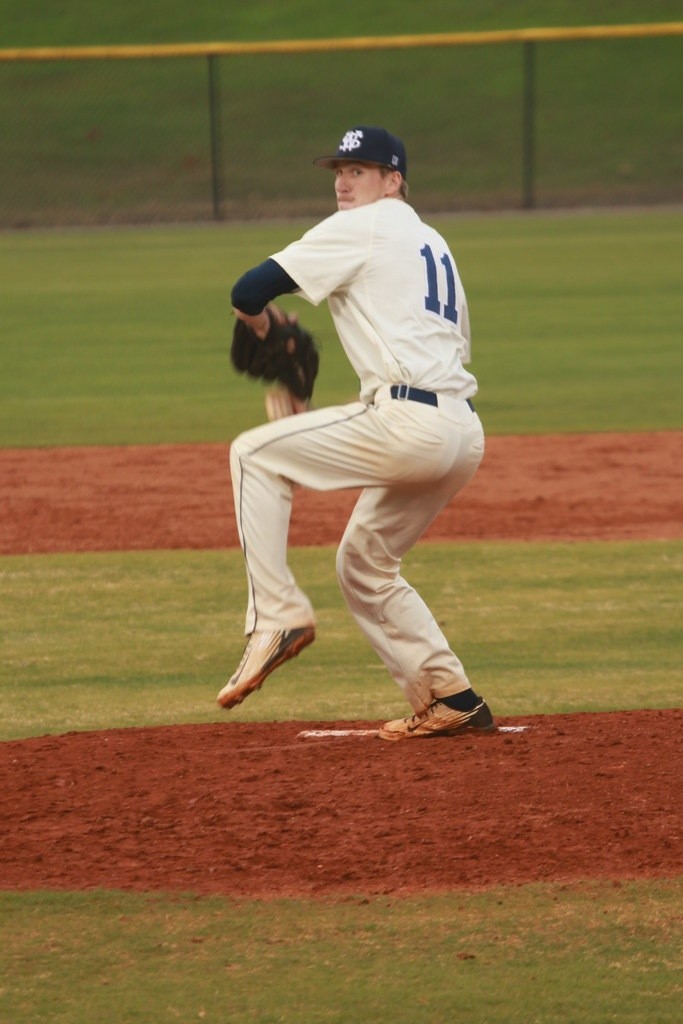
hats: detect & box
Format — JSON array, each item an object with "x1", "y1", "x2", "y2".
[{"x1": 313, "y1": 124, "x2": 407, "y2": 180}]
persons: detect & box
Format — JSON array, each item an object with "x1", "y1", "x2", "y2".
[{"x1": 216, "y1": 125, "x2": 495, "y2": 740}]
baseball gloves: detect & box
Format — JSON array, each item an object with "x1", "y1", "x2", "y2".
[{"x1": 230, "y1": 299, "x2": 321, "y2": 402}]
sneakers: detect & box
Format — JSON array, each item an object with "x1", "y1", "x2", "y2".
[
  {"x1": 217, "y1": 627, "x2": 316, "y2": 710},
  {"x1": 377, "y1": 696, "x2": 496, "y2": 741}
]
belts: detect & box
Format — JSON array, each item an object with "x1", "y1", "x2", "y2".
[{"x1": 391, "y1": 384, "x2": 475, "y2": 412}]
